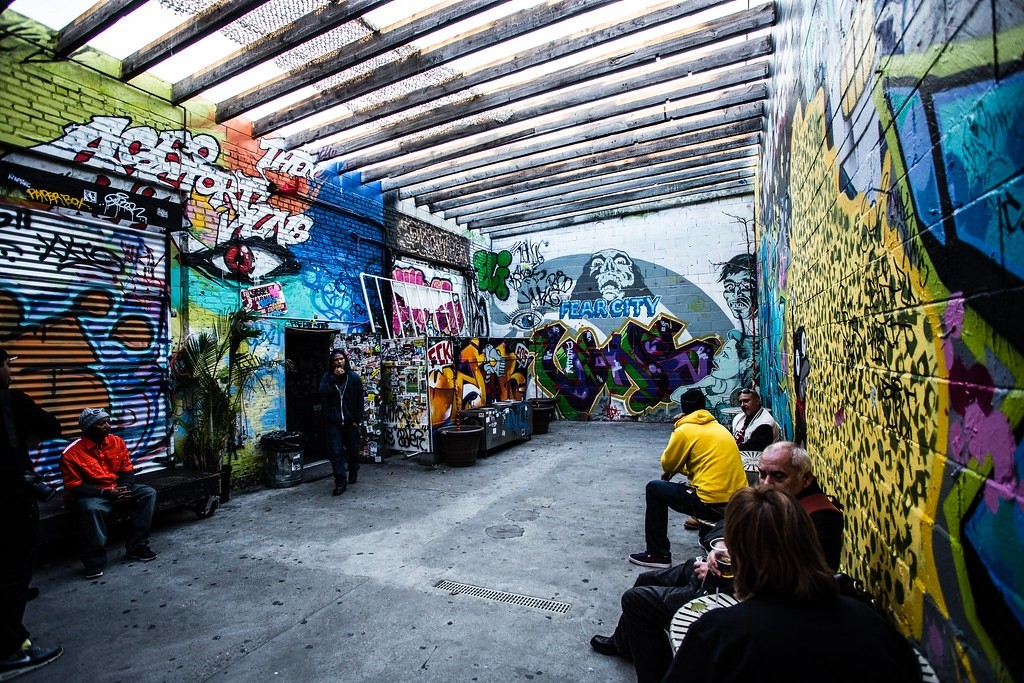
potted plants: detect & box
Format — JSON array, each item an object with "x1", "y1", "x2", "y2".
[{"x1": 166, "y1": 307, "x2": 297, "y2": 506}]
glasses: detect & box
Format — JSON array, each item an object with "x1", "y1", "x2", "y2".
[
  {"x1": 737, "y1": 389, "x2": 758, "y2": 400},
  {"x1": 83, "y1": 408, "x2": 106, "y2": 420},
  {"x1": 681, "y1": 387, "x2": 702, "y2": 403}
]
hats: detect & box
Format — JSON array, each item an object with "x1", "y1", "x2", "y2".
[{"x1": 79, "y1": 407, "x2": 110, "y2": 430}]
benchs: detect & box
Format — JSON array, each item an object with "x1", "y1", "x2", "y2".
[{"x1": 42, "y1": 469, "x2": 221, "y2": 531}]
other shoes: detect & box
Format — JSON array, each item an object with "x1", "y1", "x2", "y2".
[
  {"x1": 684, "y1": 517, "x2": 701, "y2": 528},
  {"x1": 333, "y1": 486, "x2": 346, "y2": 496},
  {"x1": 350, "y1": 472, "x2": 357, "y2": 484},
  {"x1": 27, "y1": 587, "x2": 40, "y2": 602}
]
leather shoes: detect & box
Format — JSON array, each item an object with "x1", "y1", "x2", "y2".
[{"x1": 591, "y1": 635, "x2": 621, "y2": 657}]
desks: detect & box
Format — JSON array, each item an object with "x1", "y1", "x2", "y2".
[
  {"x1": 669, "y1": 592, "x2": 942, "y2": 683},
  {"x1": 739, "y1": 451, "x2": 764, "y2": 473},
  {"x1": 458, "y1": 401, "x2": 532, "y2": 457},
  {"x1": 721, "y1": 406, "x2": 772, "y2": 434}
]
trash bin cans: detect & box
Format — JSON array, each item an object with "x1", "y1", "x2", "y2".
[{"x1": 261, "y1": 431, "x2": 303, "y2": 488}]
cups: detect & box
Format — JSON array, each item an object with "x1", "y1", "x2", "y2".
[{"x1": 710, "y1": 538, "x2": 734, "y2": 578}]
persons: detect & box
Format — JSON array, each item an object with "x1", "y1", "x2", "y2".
[
  {"x1": 589, "y1": 439, "x2": 844, "y2": 683},
  {"x1": 0, "y1": 346, "x2": 63, "y2": 681},
  {"x1": 729, "y1": 389, "x2": 780, "y2": 452},
  {"x1": 319, "y1": 348, "x2": 364, "y2": 496},
  {"x1": 666, "y1": 486, "x2": 924, "y2": 683},
  {"x1": 61, "y1": 407, "x2": 158, "y2": 578},
  {"x1": 629, "y1": 389, "x2": 749, "y2": 567}
]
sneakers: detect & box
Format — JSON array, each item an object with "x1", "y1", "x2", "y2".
[
  {"x1": 629, "y1": 551, "x2": 672, "y2": 569},
  {"x1": 82, "y1": 565, "x2": 104, "y2": 578},
  {"x1": 0, "y1": 637, "x2": 63, "y2": 682},
  {"x1": 127, "y1": 546, "x2": 158, "y2": 561}
]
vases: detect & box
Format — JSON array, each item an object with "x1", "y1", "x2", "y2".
[
  {"x1": 532, "y1": 404, "x2": 555, "y2": 433},
  {"x1": 528, "y1": 397, "x2": 559, "y2": 421},
  {"x1": 437, "y1": 425, "x2": 484, "y2": 466}
]
